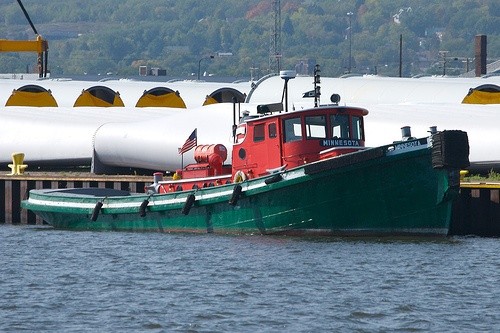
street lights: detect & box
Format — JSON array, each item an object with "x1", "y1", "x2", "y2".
[
  {"x1": 347, "y1": 11, "x2": 358, "y2": 76},
  {"x1": 197, "y1": 54, "x2": 216, "y2": 82}
]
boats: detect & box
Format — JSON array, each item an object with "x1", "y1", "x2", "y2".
[{"x1": 19, "y1": 101, "x2": 465, "y2": 241}]
